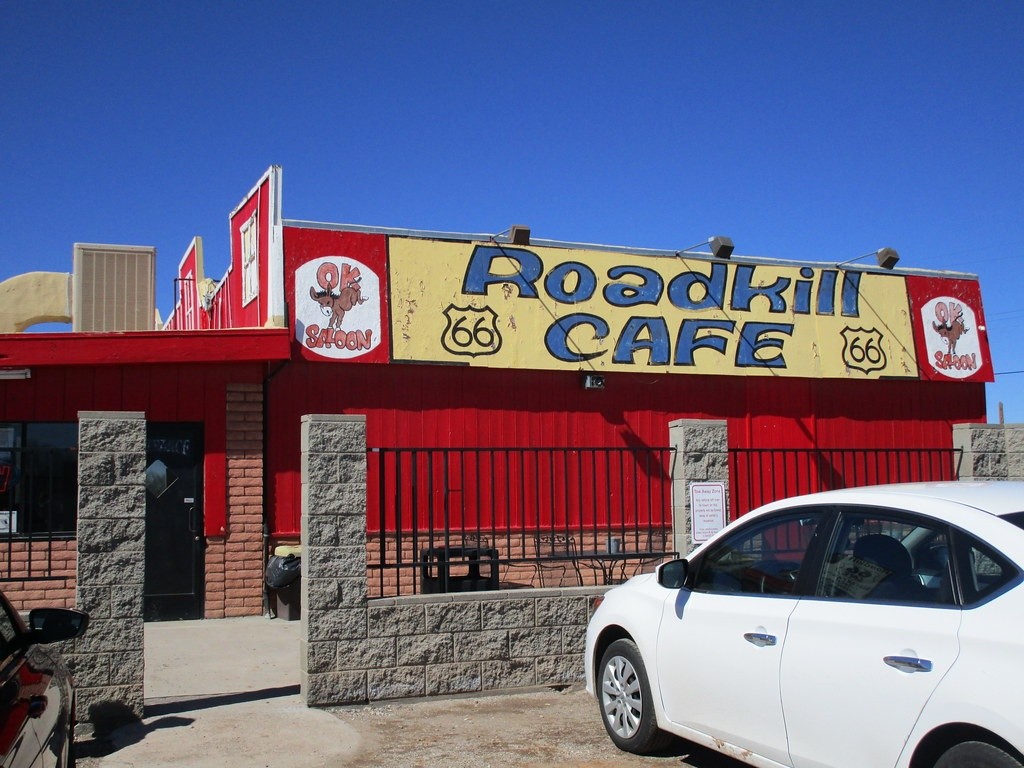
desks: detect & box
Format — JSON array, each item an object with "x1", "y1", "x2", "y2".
[{"x1": 548, "y1": 550, "x2": 663, "y2": 585}]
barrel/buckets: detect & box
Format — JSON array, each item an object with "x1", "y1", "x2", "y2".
[{"x1": 606, "y1": 538, "x2": 620, "y2": 553}]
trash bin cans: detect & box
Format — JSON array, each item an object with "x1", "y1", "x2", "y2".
[
  {"x1": 274, "y1": 546, "x2": 301, "y2": 621},
  {"x1": 420, "y1": 547, "x2": 499, "y2": 595}
]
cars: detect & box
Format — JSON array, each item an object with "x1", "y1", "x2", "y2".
[
  {"x1": 584, "y1": 481, "x2": 1024, "y2": 768},
  {"x1": 0, "y1": 592, "x2": 90, "y2": 768}
]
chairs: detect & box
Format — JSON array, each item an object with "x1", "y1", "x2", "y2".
[
  {"x1": 465, "y1": 534, "x2": 537, "y2": 590},
  {"x1": 610, "y1": 531, "x2": 670, "y2": 585},
  {"x1": 532, "y1": 534, "x2": 583, "y2": 588},
  {"x1": 829, "y1": 534, "x2": 975, "y2": 606}
]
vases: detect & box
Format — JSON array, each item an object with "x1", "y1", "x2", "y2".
[{"x1": 605, "y1": 538, "x2": 621, "y2": 553}]
